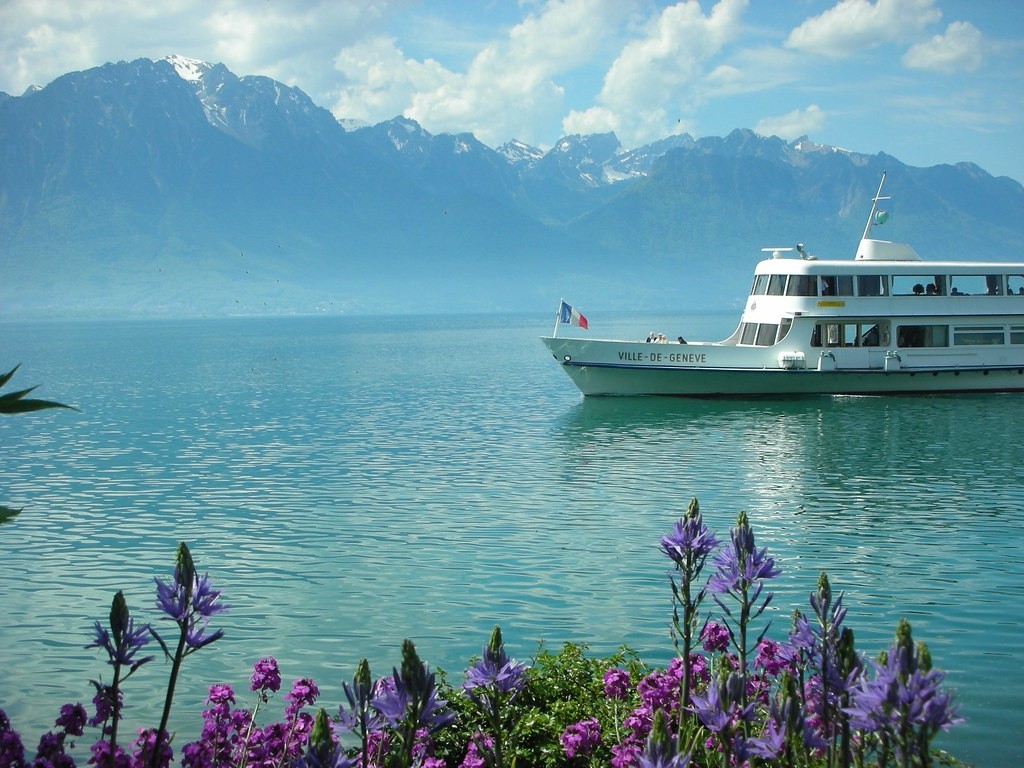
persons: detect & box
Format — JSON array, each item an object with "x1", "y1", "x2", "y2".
[
  {"x1": 678, "y1": 336, "x2": 687, "y2": 344},
  {"x1": 951, "y1": 287, "x2": 962, "y2": 295},
  {"x1": 646, "y1": 332, "x2": 669, "y2": 344},
  {"x1": 1019, "y1": 287, "x2": 1023, "y2": 295}
]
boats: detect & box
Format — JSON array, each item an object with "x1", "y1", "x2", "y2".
[{"x1": 538, "y1": 170, "x2": 1024, "y2": 397}]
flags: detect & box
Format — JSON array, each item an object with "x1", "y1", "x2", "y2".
[{"x1": 559, "y1": 302, "x2": 588, "y2": 329}]
